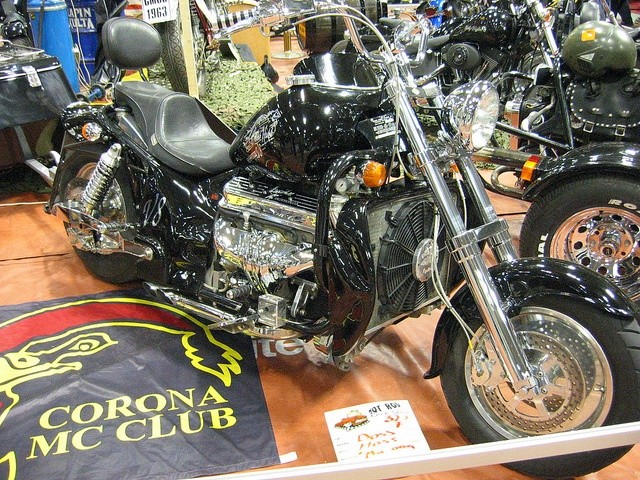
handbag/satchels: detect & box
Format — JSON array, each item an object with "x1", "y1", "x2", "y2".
[
  {"x1": 198, "y1": 45, "x2": 280, "y2": 129},
  {"x1": 564, "y1": 68, "x2": 640, "y2": 142}
]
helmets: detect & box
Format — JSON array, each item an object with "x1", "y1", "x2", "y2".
[
  {"x1": 277, "y1": 53, "x2": 397, "y2": 183},
  {"x1": 562, "y1": 20, "x2": 638, "y2": 78}
]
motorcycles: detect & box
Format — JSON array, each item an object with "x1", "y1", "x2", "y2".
[
  {"x1": 287, "y1": 1, "x2": 639, "y2": 310},
  {"x1": 43, "y1": 0, "x2": 640, "y2": 476}
]
